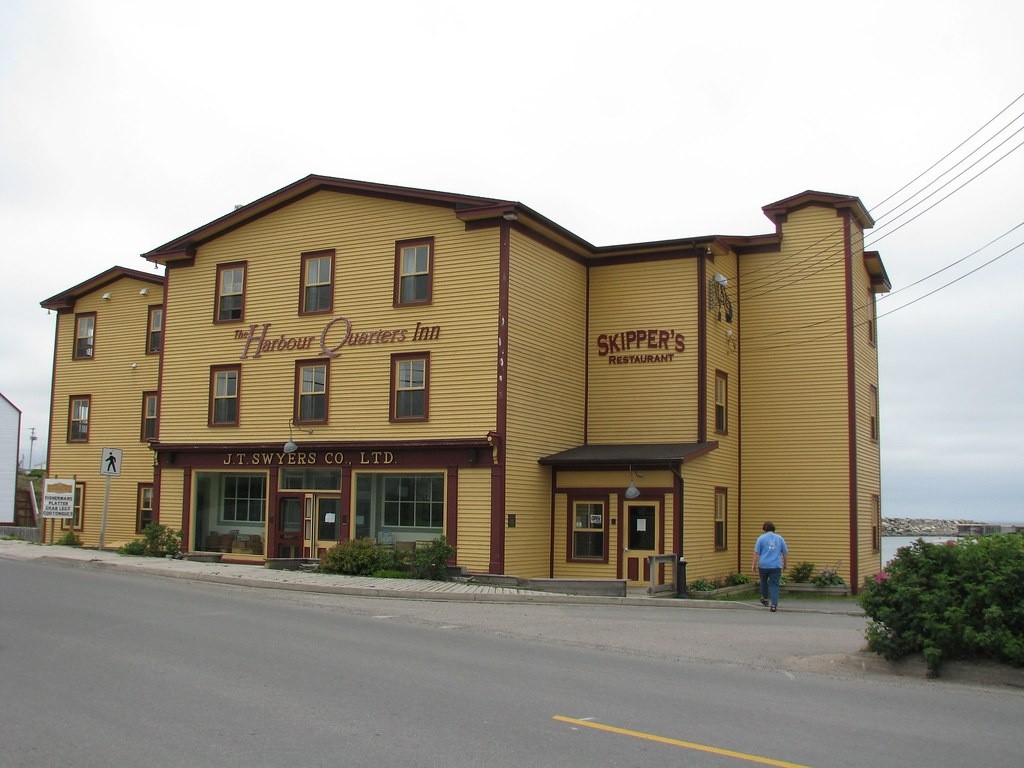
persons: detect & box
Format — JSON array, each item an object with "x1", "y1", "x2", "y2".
[{"x1": 752, "y1": 522, "x2": 789, "y2": 612}]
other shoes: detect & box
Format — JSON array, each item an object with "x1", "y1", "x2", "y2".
[
  {"x1": 761, "y1": 597, "x2": 770, "y2": 607},
  {"x1": 770, "y1": 605, "x2": 777, "y2": 612}
]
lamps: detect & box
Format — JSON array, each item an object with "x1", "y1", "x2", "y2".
[
  {"x1": 283, "y1": 417, "x2": 314, "y2": 453},
  {"x1": 624, "y1": 464, "x2": 644, "y2": 500}
]
